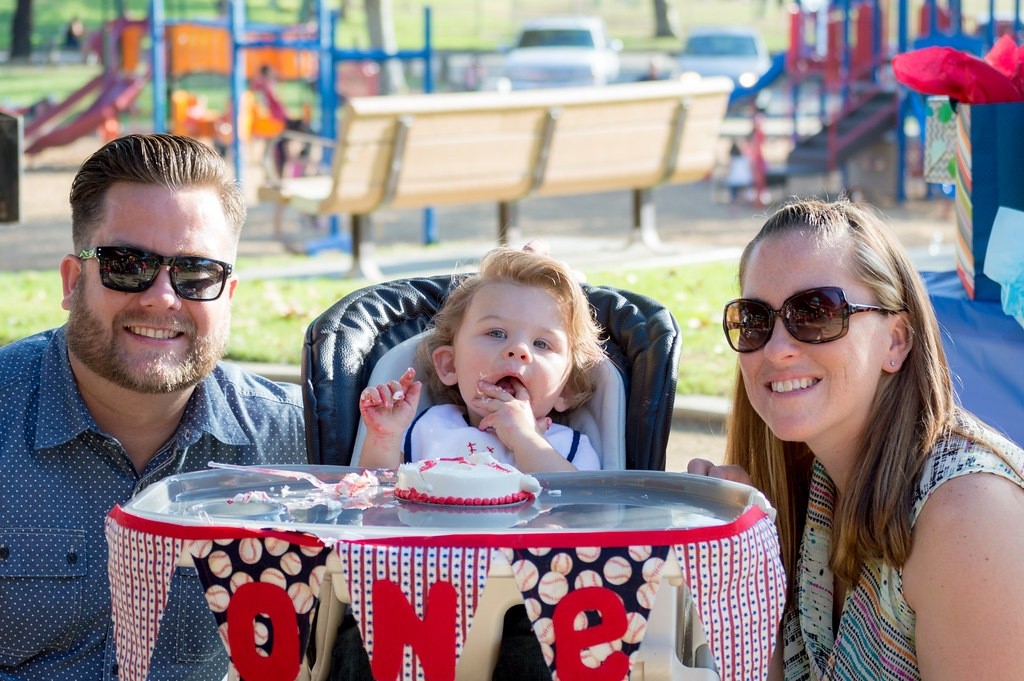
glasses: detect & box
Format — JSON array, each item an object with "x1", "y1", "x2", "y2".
[
  {"x1": 78, "y1": 246, "x2": 232, "y2": 301},
  {"x1": 722, "y1": 287, "x2": 908, "y2": 353}
]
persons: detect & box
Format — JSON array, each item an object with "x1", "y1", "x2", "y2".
[
  {"x1": 60, "y1": 16, "x2": 85, "y2": 51},
  {"x1": 171, "y1": 265, "x2": 179, "y2": 281},
  {"x1": 679, "y1": 201, "x2": 1024, "y2": 681},
  {"x1": 723, "y1": 143, "x2": 753, "y2": 202},
  {"x1": 823, "y1": 308, "x2": 833, "y2": 325},
  {"x1": 252, "y1": 62, "x2": 314, "y2": 180},
  {"x1": 0, "y1": 133, "x2": 311, "y2": 681},
  {"x1": 100, "y1": 254, "x2": 147, "y2": 277},
  {"x1": 359, "y1": 250, "x2": 624, "y2": 473}
]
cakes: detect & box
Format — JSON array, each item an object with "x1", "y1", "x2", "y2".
[
  {"x1": 333, "y1": 470, "x2": 381, "y2": 494},
  {"x1": 394, "y1": 450, "x2": 543, "y2": 505}
]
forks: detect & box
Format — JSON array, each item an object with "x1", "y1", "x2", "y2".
[{"x1": 208, "y1": 461, "x2": 372, "y2": 491}]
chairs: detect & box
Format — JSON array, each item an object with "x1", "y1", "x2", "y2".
[{"x1": 301, "y1": 273, "x2": 682, "y2": 472}]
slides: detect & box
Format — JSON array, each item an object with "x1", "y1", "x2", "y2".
[
  {"x1": 730, "y1": 51, "x2": 789, "y2": 103},
  {"x1": 24, "y1": 67, "x2": 149, "y2": 154}
]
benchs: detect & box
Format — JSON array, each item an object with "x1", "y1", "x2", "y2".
[{"x1": 259, "y1": 76, "x2": 736, "y2": 281}]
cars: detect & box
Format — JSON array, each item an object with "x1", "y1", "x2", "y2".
[
  {"x1": 674, "y1": 27, "x2": 773, "y2": 118},
  {"x1": 495, "y1": 15, "x2": 624, "y2": 91}
]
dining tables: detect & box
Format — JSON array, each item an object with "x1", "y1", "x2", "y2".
[{"x1": 104, "y1": 465, "x2": 789, "y2": 681}]
